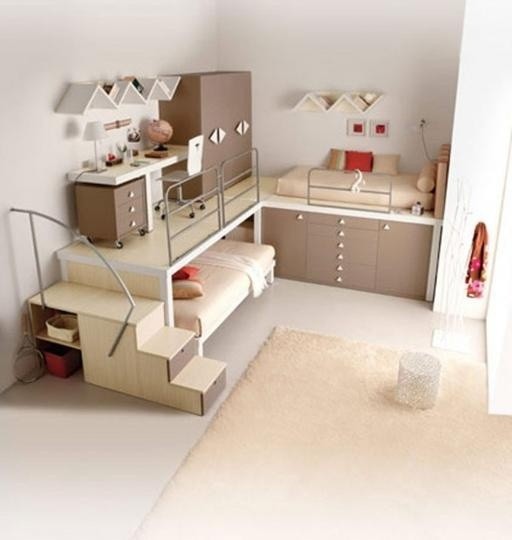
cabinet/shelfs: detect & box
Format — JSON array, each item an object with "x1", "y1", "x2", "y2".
[
  {"x1": 376, "y1": 220, "x2": 433, "y2": 302},
  {"x1": 260, "y1": 206, "x2": 306, "y2": 283},
  {"x1": 114, "y1": 177, "x2": 147, "y2": 240},
  {"x1": 201, "y1": 73, "x2": 252, "y2": 199},
  {"x1": 307, "y1": 212, "x2": 378, "y2": 296}
]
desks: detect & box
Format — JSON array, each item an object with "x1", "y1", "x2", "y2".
[{"x1": 69, "y1": 142, "x2": 189, "y2": 233}]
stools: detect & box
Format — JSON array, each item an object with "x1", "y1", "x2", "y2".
[{"x1": 397, "y1": 351, "x2": 441, "y2": 410}]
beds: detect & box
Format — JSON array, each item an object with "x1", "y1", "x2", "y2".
[
  {"x1": 276, "y1": 144, "x2": 450, "y2": 219},
  {"x1": 173, "y1": 240, "x2": 276, "y2": 357}
]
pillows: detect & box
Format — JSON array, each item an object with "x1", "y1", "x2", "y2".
[
  {"x1": 172, "y1": 279, "x2": 203, "y2": 299},
  {"x1": 171, "y1": 266, "x2": 199, "y2": 278},
  {"x1": 373, "y1": 153, "x2": 400, "y2": 175},
  {"x1": 346, "y1": 150, "x2": 372, "y2": 173},
  {"x1": 416, "y1": 167, "x2": 435, "y2": 192},
  {"x1": 327, "y1": 148, "x2": 344, "y2": 170}
]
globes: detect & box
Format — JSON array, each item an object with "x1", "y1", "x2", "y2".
[{"x1": 148, "y1": 120, "x2": 173, "y2": 151}]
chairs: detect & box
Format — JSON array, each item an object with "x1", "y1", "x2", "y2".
[{"x1": 152, "y1": 135, "x2": 205, "y2": 219}]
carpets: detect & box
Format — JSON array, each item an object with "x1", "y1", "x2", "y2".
[{"x1": 130, "y1": 324, "x2": 512, "y2": 538}]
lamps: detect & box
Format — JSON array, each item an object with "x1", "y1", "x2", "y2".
[{"x1": 83, "y1": 121, "x2": 107, "y2": 173}]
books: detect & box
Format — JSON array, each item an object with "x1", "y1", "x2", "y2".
[
  {"x1": 124, "y1": 76, "x2": 145, "y2": 93},
  {"x1": 103, "y1": 82, "x2": 119, "y2": 101},
  {"x1": 160, "y1": 80, "x2": 170, "y2": 93}
]
